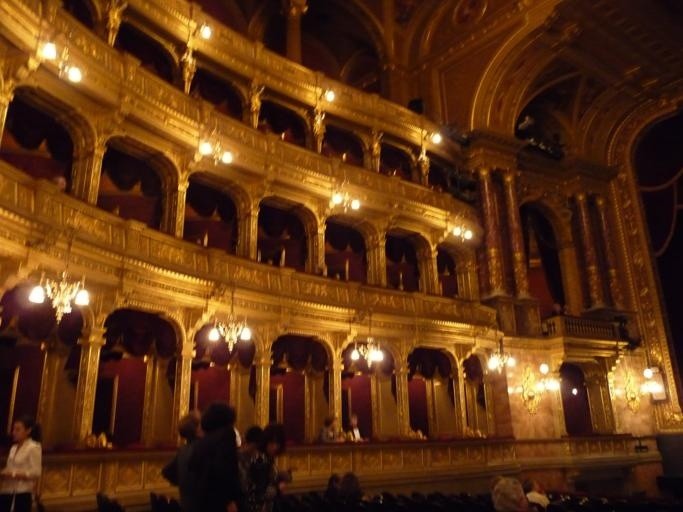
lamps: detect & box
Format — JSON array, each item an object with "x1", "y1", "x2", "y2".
[
  {"x1": 27, "y1": 239, "x2": 90, "y2": 326},
  {"x1": 208, "y1": 285, "x2": 250, "y2": 354},
  {"x1": 191, "y1": 20, "x2": 212, "y2": 39},
  {"x1": 197, "y1": 128, "x2": 233, "y2": 166},
  {"x1": 422, "y1": 129, "x2": 443, "y2": 147},
  {"x1": 332, "y1": 181, "x2": 361, "y2": 213},
  {"x1": 42, "y1": 24, "x2": 82, "y2": 84},
  {"x1": 454, "y1": 218, "x2": 473, "y2": 244},
  {"x1": 486, "y1": 325, "x2": 517, "y2": 374},
  {"x1": 318, "y1": 84, "x2": 334, "y2": 102},
  {"x1": 357, "y1": 307, "x2": 380, "y2": 368}
]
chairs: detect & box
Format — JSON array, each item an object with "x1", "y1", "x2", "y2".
[{"x1": 96, "y1": 490, "x2": 682, "y2": 512}]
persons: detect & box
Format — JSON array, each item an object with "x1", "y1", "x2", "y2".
[
  {"x1": 318, "y1": 416, "x2": 339, "y2": 443},
  {"x1": 0, "y1": 417, "x2": 43, "y2": 512},
  {"x1": 491, "y1": 478, "x2": 529, "y2": 512},
  {"x1": 161, "y1": 404, "x2": 280, "y2": 512}
]
